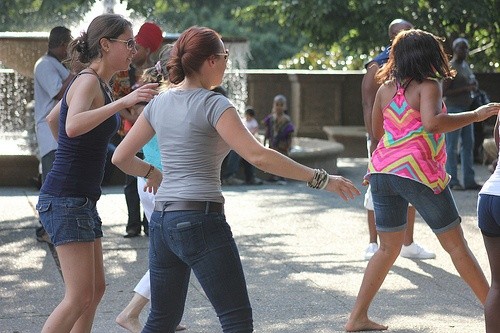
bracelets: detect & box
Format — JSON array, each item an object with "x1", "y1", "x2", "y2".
[
  {"x1": 145, "y1": 163, "x2": 154, "y2": 179},
  {"x1": 306, "y1": 168, "x2": 330, "y2": 191},
  {"x1": 474, "y1": 109, "x2": 480, "y2": 122}
]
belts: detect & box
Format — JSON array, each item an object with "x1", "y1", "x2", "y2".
[{"x1": 154, "y1": 201, "x2": 223, "y2": 212}]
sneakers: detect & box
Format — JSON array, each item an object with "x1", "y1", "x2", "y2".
[
  {"x1": 365, "y1": 242, "x2": 378, "y2": 260},
  {"x1": 400, "y1": 241, "x2": 436, "y2": 259}
]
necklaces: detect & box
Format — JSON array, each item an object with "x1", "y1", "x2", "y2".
[{"x1": 88, "y1": 66, "x2": 115, "y2": 103}]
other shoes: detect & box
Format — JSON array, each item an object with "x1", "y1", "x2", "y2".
[
  {"x1": 246, "y1": 176, "x2": 262, "y2": 185},
  {"x1": 144, "y1": 226, "x2": 149, "y2": 236},
  {"x1": 452, "y1": 184, "x2": 464, "y2": 191},
  {"x1": 465, "y1": 182, "x2": 482, "y2": 189},
  {"x1": 124, "y1": 231, "x2": 136, "y2": 237},
  {"x1": 263, "y1": 176, "x2": 286, "y2": 185},
  {"x1": 223, "y1": 177, "x2": 245, "y2": 186}
]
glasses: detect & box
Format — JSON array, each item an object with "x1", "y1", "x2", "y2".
[
  {"x1": 215, "y1": 49, "x2": 229, "y2": 60},
  {"x1": 109, "y1": 38, "x2": 136, "y2": 50}
]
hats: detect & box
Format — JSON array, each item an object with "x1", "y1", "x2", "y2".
[{"x1": 135, "y1": 23, "x2": 163, "y2": 53}]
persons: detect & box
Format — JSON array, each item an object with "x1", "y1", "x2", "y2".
[
  {"x1": 344, "y1": 30, "x2": 500, "y2": 332},
  {"x1": 34, "y1": 26, "x2": 294, "y2": 333},
  {"x1": 477, "y1": 109, "x2": 500, "y2": 333},
  {"x1": 363, "y1": 19, "x2": 490, "y2": 260},
  {"x1": 35, "y1": 13, "x2": 159, "y2": 332},
  {"x1": 111, "y1": 27, "x2": 361, "y2": 333}
]
